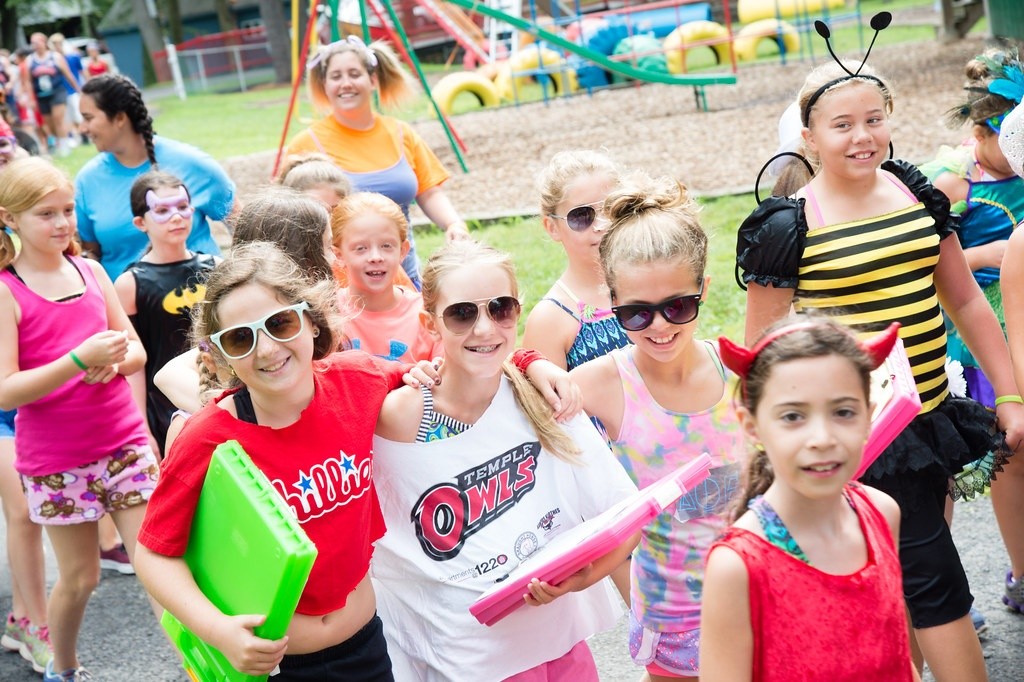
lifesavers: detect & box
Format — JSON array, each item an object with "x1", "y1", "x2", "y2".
[
  {"x1": 432, "y1": 72, "x2": 499, "y2": 119},
  {"x1": 609, "y1": 35, "x2": 666, "y2": 84},
  {"x1": 571, "y1": 16, "x2": 671, "y2": 88},
  {"x1": 734, "y1": 19, "x2": 802, "y2": 66},
  {"x1": 663, "y1": 19, "x2": 732, "y2": 73},
  {"x1": 496, "y1": 47, "x2": 576, "y2": 107}
]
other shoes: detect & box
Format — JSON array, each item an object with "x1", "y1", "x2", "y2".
[{"x1": 968, "y1": 607, "x2": 987, "y2": 636}]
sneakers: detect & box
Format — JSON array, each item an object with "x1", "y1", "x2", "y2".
[
  {"x1": 1, "y1": 614, "x2": 30, "y2": 651},
  {"x1": 42, "y1": 658, "x2": 94, "y2": 682},
  {"x1": 99, "y1": 541, "x2": 135, "y2": 575},
  {"x1": 998, "y1": 570, "x2": 1024, "y2": 611},
  {"x1": 20, "y1": 622, "x2": 56, "y2": 674}
]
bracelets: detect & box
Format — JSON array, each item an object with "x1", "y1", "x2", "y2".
[
  {"x1": 510, "y1": 348, "x2": 548, "y2": 375},
  {"x1": 995, "y1": 395, "x2": 1023, "y2": 406},
  {"x1": 69, "y1": 352, "x2": 87, "y2": 370}
]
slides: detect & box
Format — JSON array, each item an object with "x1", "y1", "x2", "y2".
[{"x1": 448, "y1": 0, "x2": 738, "y2": 86}]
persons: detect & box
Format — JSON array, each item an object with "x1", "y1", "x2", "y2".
[{"x1": 0, "y1": 32, "x2": 1024, "y2": 682}]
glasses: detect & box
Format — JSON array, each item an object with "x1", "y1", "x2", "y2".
[
  {"x1": 430, "y1": 295, "x2": 522, "y2": 334},
  {"x1": 210, "y1": 300, "x2": 309, "y2": 360},
  {"x1": 548, "y1": 200, "x2": 611, "y2": 232},
  {"x1": 611, "y1": 278, "x2": 704, "y2": 331}
]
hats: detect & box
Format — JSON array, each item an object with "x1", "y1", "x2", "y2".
[
  {"x1": 998, "y1": 90, "x2": 1024, "y2": 181},
  {"x1": 769, "y1": 102, "x2": 805, "y2": 175}
]
